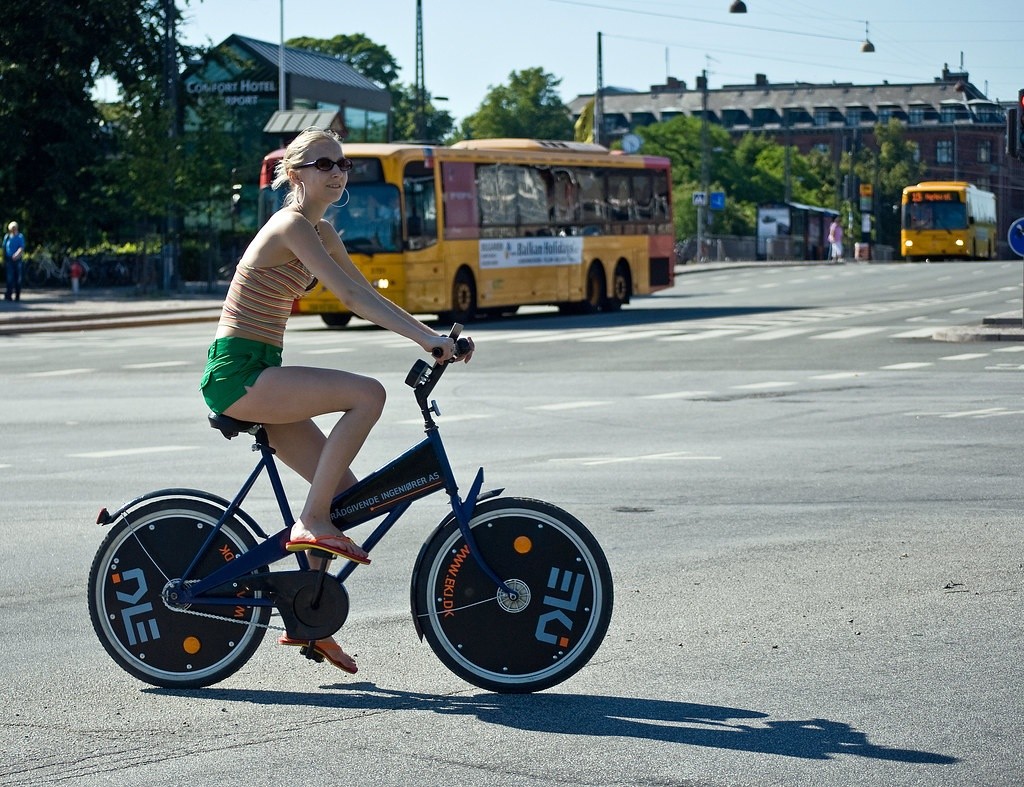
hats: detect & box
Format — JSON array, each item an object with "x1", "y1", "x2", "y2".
[{"x1": 7, "y1": 221, "x2": 18, "y2": 230}]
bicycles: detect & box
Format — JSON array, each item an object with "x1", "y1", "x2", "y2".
[
  {"x1": 22, "y1": 246, "x2": 136, "y2": 289},
  {"x1": 86, "y1": 321, "x2": 615, "y2": 696}
]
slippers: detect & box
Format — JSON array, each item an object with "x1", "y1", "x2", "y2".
[
  {"x1": 278, "y1": 632, "x2": 359, "y2": 674},
  {"x1": 285, "y1": 535, "x2": 372, "y2": 565}
]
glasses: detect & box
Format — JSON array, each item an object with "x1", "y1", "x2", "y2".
[{"x1": 292, "y1": 156, "x2": 355, "y2": 172}]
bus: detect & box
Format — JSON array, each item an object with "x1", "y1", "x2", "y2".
[
  {"x1": 231, "y1": 134, "x2": 677, "y2": 328},
  {"x1": 892, "y1": 181, "x2": 1000, "y2": 262}
]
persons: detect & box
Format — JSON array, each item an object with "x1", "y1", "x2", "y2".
[
  {"x1": 828, "y1": 214, "x2": 845, "y2": 262},
  {"x1": 205, "y1": 130, "x2": 476, "y2": 674},
  {"x1": 0, "y1": 222, "x2": 27, "y2": 301}
]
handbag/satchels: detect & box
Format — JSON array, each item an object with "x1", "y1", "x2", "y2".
[{"x1": 0, "y1": 246, "x2": 4, "y2": 263}]
